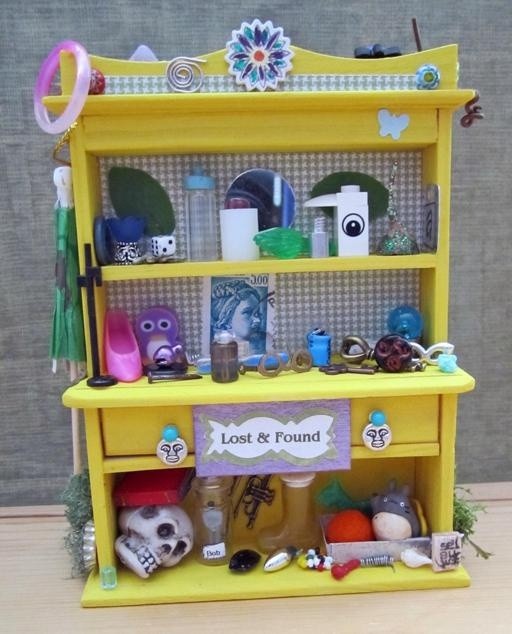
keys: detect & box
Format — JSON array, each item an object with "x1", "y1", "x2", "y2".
[{"x1": 320, "y1": 362, "x2": 375, "y2": 375}]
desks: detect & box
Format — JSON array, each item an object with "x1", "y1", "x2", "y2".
[{"x1": 63, "y1": 363, "x2": 475, "y2": 412}]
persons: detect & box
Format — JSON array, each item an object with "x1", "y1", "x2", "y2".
[{"x1": 210, "y1": 280, "x2": 267, "y2": 358}]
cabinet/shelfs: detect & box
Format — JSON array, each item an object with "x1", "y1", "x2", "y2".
[
  {"x1": 42, "y1": 85, "x2": 476, "y2": 365},
  {"x1": 80, "y1": 392, "x2": 472, "y2": 609}
]
